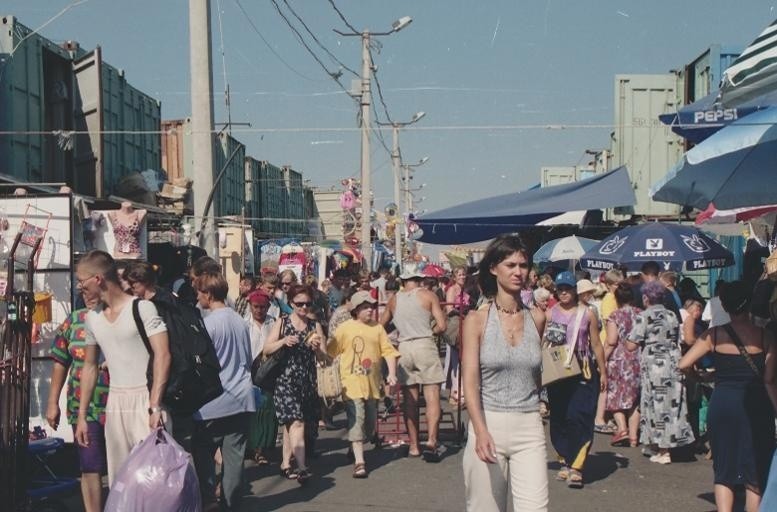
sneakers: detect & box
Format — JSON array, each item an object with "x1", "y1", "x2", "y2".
[
  {"x1": 648, "y1": 452, "x2": 672, "y2": 464},
  {"x1": 641, "y1": 446, "x2": 657, "y2": 456}
]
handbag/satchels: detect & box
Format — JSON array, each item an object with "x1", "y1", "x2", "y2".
[
  {"x1": 541, "y1": 308, "x2": 584, "y2": 388},
  {"x1": 133, "y1": 300, "x2": 222, "y2": 418},
  {"x1": 316, "y1": 354, "x2": 341, "y2": 409},
  {"x1": 253, "y1": 320, "x2": 287, "y2": 388}
]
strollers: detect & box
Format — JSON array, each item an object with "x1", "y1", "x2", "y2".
[{"x1": 27, "y1": 430, "x2": 78, "y2": 502}]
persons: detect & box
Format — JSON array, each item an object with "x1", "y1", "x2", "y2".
[{"x1": 45, "y1": 237, "x2": 776, "y2": 512}]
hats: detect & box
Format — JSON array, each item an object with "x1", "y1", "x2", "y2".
[
  {"x1": 348, "y1": 291, "x2": 379, "y2": 312},
  {"x1": 554, "y1": 270, "x2": 577, "y2": 289},
  {"x1": 575, "y1": 279, "x2": 596, "y2": 297},
  {"x1": 398, "y1": 264, "x2": 426, "y2": 280}
]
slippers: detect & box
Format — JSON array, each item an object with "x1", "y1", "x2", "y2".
[
  {"x1": 423, "y1": 445, "x2": 440, "y2": 463},
  {"x1": 557, "y1": 466, "x2": 583, "y2": 488}
]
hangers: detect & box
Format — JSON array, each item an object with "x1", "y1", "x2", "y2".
[{"x1": 290, "y1": 238, "x2": 297, "y2": 246}]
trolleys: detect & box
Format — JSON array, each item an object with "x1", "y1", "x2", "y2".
[{"x1": 369, "y1": 282, "x2": 466, "y2": 453}]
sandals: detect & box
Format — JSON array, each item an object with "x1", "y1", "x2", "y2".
[
  {"x1": 297, "y1": 463, "x2": 313, "y2": 483},
  {"x1": 254, "y1": 451, "x2": 270, "y2": 469},
  {"x1": 449, "y1": 390, "x2": 467, "y2": 411},
  {"x1": 279, "y1": 464, "x2": 297, "y2": 479},
  {"x1": 594, "y1": 424, "x2": 638, "y2": 449},
  {"x1": 347, "y1": 445, "x2": 369, "y2": 478}
]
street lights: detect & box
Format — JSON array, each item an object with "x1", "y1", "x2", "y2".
[
  {"x1": 401, "y1": 196, "x2": 425, "y2": 216},
  {"x1": 400, "y1": 183, "x2": 426, "y2": 239},
  {"x1": 330, "y1": 15, "x2": 411, "y2": 277},
  {"x1": 373, "y1": 110, "x2": 424, "y2": 272},
  {"x1": 399, "y1": 156, "x2": 429, "y2": 257}
]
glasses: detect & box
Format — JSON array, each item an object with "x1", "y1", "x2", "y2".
[
  {"x1": 78, "y1": 275, "x2": 96, "y2": 286},
  {"x1": 292, "y1": 300, "x2": 312, "y2": 308}
]
